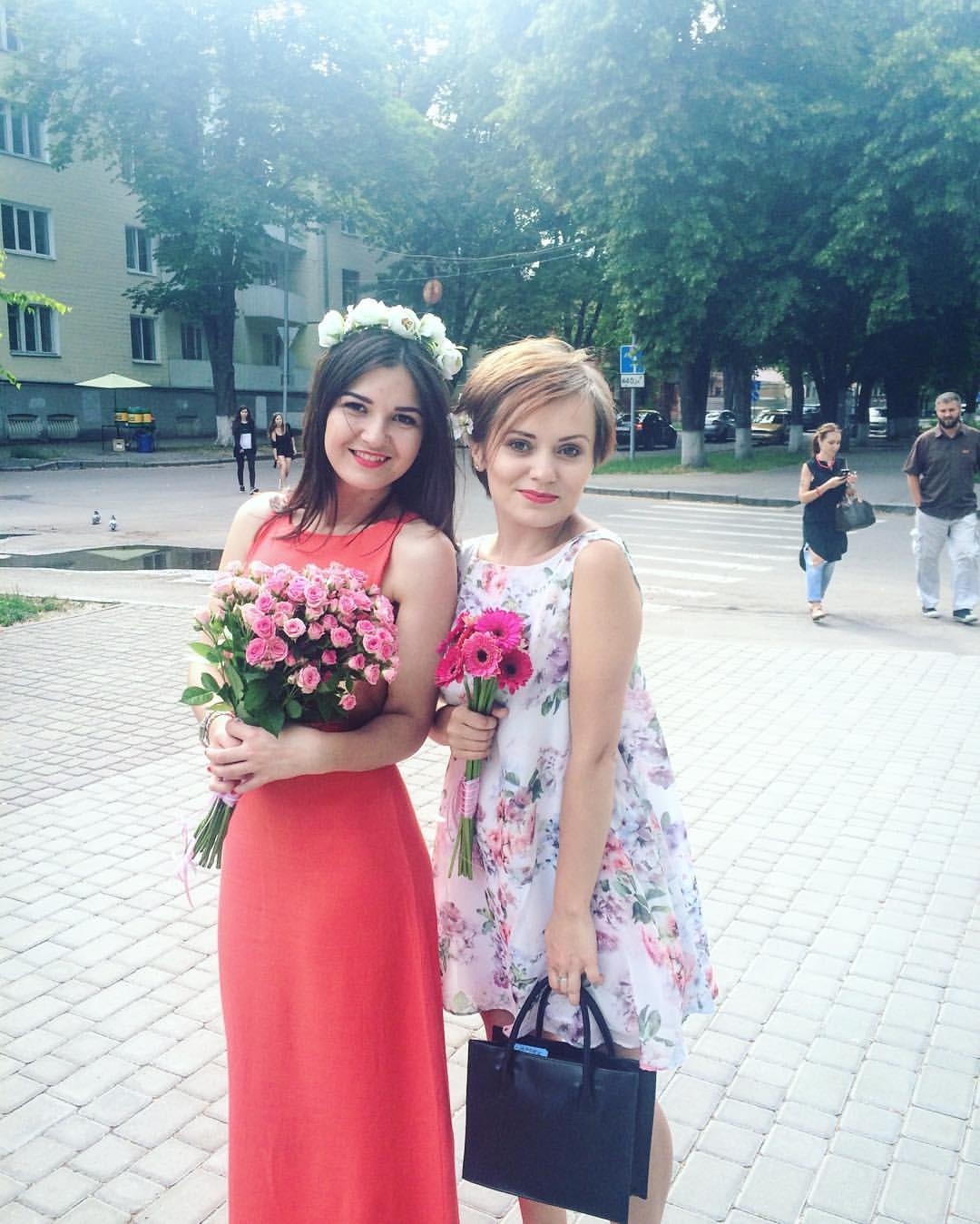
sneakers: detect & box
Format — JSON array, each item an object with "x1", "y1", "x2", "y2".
[
  {"x1": 953, "y1": 608, "x2": 978, "y2": 623},
  {"x1": 922, "y1": 605, "x2": 939, "y2": 618}
]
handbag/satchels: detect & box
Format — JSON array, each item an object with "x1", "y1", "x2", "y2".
[
  {"x1": 461, "y1": 976, "x2": 657, "y2": 1224},
  {"x1": 832, "y1": 491, "x2": 875, "y2": 531}
]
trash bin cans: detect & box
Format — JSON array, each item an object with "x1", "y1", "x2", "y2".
[
  {"x1": 112, "y1": 436, "x2": 125, "y2": 452},
  {"x1": 137, "y1": 432, "x2": 154, "y2": 453}
]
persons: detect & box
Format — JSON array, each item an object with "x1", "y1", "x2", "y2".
[
  {"x1": 902, "y1": 392, "x2": 980, "y2": 623},
  {"x1": 188, "y1": 321, "x2": 459, "y2": 1224},
  {"x1": 269, "y1": 412, "x2": 297, "y2": 490},
  {"x1": 232, "y1": 405, "x2": 260, "y2": 495},
  {"x1": 798, "y1": 423, "x2": 857, "y2": 621},
  {"x1": 436, "y1": 335, "x2": 719, "y2": 1224}
]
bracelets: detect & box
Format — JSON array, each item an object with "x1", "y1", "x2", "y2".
[{"x1": 816, "y1": 486, "x2": 823, "y2": 497}]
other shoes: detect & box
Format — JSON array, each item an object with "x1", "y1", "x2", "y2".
[
  {"x1": 249, "y1": 487, "x2": 258, "y2": 494},
  {"x1": 812, "y1": 608, "x2": 828, "y2": 621},
  {"x1": 239, "y1": 486, "x2": 245, "y2": 493}
]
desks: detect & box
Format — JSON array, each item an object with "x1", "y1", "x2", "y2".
[{"x1": 101, "y1": 424, "x2": 157, "y2": 451}]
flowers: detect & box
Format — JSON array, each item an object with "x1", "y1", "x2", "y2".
[
  {"x1": 173, "y1": 560, "x2": 401, "y2": 871},
  {"x1": 429, "y1": 605, "x2": 536, "y2": 881},
  {"x1": 447, "y1": 392, "x2": 474, "y2": 443},
  {"x1": 316, "y1": 295, "x2": 468, "y2": 382}
]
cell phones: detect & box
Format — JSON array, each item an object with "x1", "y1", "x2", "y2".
[{"x1": 839, "y1": 469, "x2": 850, "y2": 477}]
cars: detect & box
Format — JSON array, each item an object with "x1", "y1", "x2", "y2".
[
  {"x1": 869, "y1": 407, "x2": 888, "y2": 438},
  {"x1": 751, "y1": 409, "x2": 791, "y2": 444},
  {"x1": 613, "y1": 409, "x2": 677, "y2": 450},
  {"x1": 802, "y1": 403, "x2": 824, "y2": 433},
  {"x1": 704, "y1": 409, "x2": 736, "y2": 442}
]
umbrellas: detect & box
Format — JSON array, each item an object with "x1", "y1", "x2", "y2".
[{"x1": 75, "y1": 373, "x2": 153, "y2": 437}]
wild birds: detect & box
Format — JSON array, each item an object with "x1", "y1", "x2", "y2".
[{"x1": 92, "y1": 510, "x2": 118, "y2": 532}]
